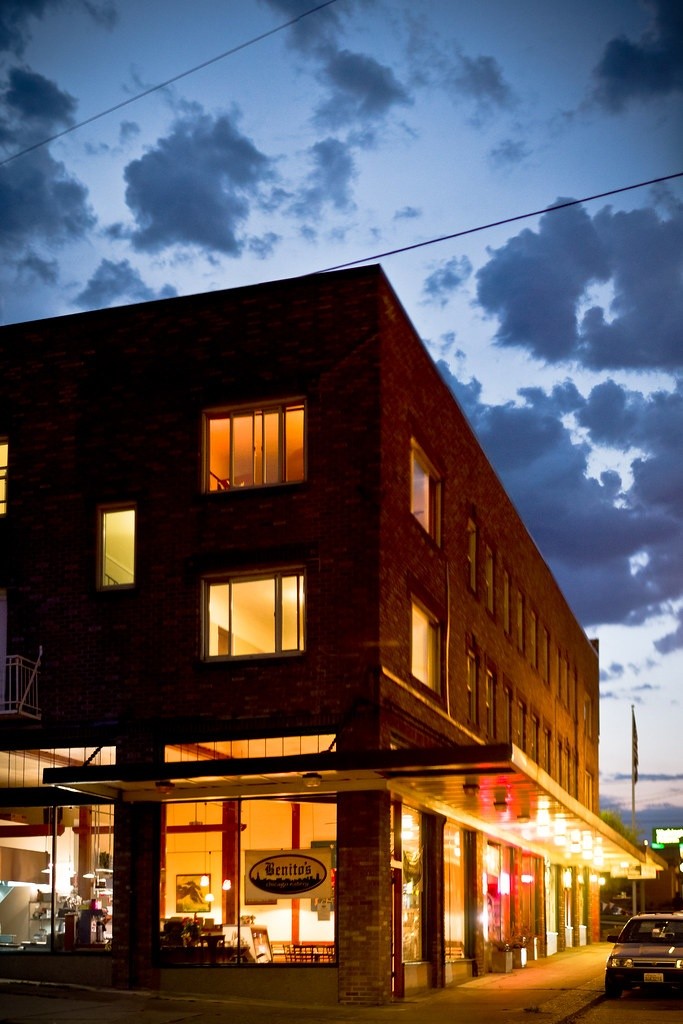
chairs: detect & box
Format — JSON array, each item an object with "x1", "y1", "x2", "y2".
[{"x1": 282, "y1": 944, "x2": 336, "y2": 963}]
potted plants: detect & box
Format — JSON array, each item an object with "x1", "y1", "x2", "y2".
[{"x1": 489, "y1": 931, "x2": 530, "y2": 973}]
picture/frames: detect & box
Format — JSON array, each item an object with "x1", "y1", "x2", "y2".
[{"x1": 176, "y1": 873, "x2": 211, "y2": 913}]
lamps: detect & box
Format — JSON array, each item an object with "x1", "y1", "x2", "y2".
[
  {"x1": 463, "y1": 784, "x2": 481, "y2": 799},
  {"x1": 516, "y1": 815, "x2": 531, "y2": 823},
  {"x1": 155, "y1": 781, "x2": 176, "y2": 794},
  {"x1": 40, "y1": 808, "x2": 53, "y2": 874},
  {"x1": 83, "y1": 811, "x2": 98, "y2": 879},
  {"x1": 493, "y1": 801, "x2": 508, "y2": 812},
  {"x1": 303, "y1": 772, "x2": 323, "y2": 788}
]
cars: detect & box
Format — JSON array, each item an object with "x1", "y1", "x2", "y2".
[{"x1": 604, "y1": 910, "x2": 683, "y2": 1000}]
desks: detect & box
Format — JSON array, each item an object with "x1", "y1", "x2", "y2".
[
  {"x1": 293, "y1": 943, "x2": 333, "y2": 963},
  {"x1": 200, "y1": 933, "x2": 226, "y2": 959}
]
